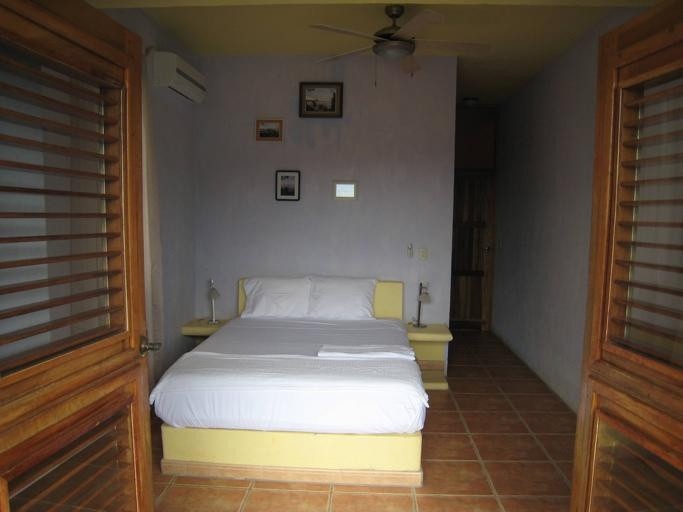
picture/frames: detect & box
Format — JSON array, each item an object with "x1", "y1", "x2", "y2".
[
  {"x1": 275, "y1": 170, "x2": 300, "y2": 201},
  {"x1": 299, "y1": 82, "x2": 343, "y2": 119},
  {"x1": 255, "y1": 118, "x2": 283, "y2": 142}
]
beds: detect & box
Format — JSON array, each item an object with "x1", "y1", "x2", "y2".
[{"x1": 149, "y1": 279, "x2": 430, "y2": 488}]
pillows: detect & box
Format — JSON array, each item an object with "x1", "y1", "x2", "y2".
[{"x1": 246, "y1": 275, "x2": 377, "y2": 322}]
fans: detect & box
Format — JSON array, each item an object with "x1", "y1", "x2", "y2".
[{"x1": 309, "y1": 4, "x2": 486, "y2": 67}]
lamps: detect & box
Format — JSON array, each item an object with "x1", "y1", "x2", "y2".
[{"x1": 412, "y1": 280, "x2": 430, "y2": 327}]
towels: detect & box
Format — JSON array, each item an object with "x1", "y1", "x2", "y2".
[{"x1": 317, "y1": 343, "x2": 416, "y2": 359}]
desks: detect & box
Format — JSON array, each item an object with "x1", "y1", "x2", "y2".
[
  {"x1": 178, "y1": 319, "x2": 229, "y2": 348},
  {"x1": 406, "y1": 323, "x2": 454, "y2": 389}
]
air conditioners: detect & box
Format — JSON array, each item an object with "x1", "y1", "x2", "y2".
[{"x1": 147, "y1": 51, "x2": 208, "y2": 106}]
